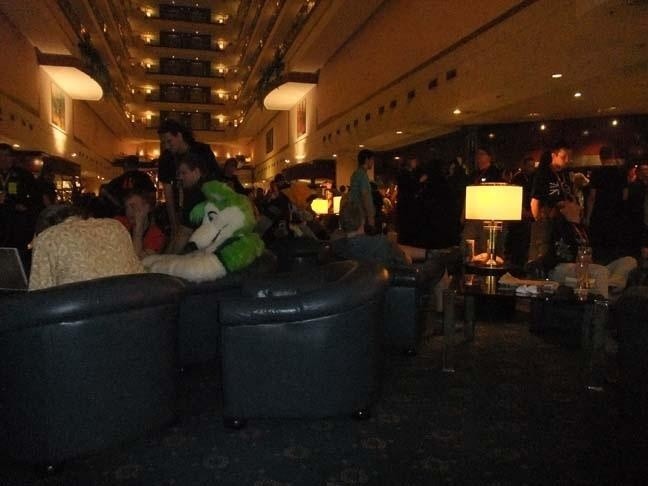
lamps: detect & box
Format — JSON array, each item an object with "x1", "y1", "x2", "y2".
[
  {"x1": 39, "y1": 53, "x2": 104, "y2": 103},
  {"x1": 464, "y1": 185, "x2": 523, "y2": 273},
  {"x1": 261, "y1": 73, "x2": 317, "y2": 111}
]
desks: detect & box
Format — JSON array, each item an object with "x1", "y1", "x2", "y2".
[{"x1": 459, "y1": 286, "x2": 595, "y2": 329}]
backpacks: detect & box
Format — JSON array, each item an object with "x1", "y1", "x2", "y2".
[{"x1": 338, "y1": 192, "x2": 362, "y2": 233}]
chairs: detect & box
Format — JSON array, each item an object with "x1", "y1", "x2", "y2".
[
  {"x1": 286, "y1": 240, "x2": 418, "y2": 357},
  {"x1": 0, "y1": 273, "x2": 192, "y2": 485},
  {"x1": 615, "y1": 266, "x2": 648, "y2": 422},
  {"x1": 215, "y1": 257, "x2": 389, "y2": 433}
]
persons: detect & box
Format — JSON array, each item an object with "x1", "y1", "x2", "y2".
[{"x1": 0, "y1": 115, "x2": 648, "y2": 341}]
